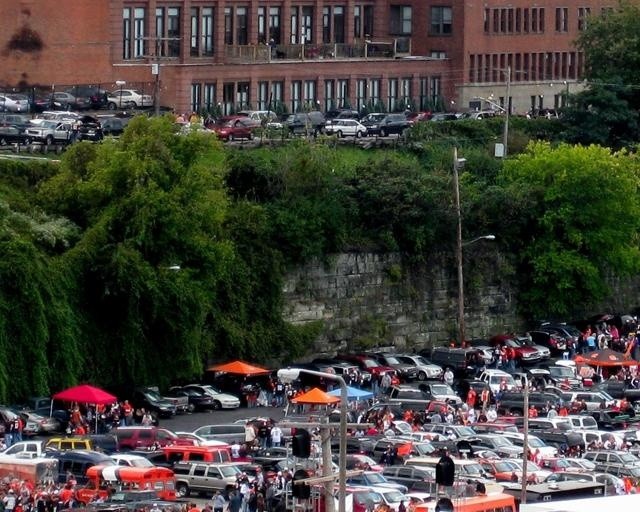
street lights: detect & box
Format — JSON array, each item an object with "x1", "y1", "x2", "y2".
[{"x1": 455, "y1": 234, "x2": 497, "y2": 350}]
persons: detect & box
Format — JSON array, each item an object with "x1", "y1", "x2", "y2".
[{"x1": 171, "y1": 109, "x2": 199, "y2": 124}]
